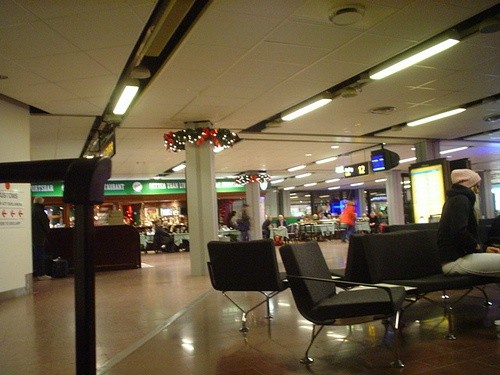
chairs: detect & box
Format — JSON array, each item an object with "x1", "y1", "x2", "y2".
[
  {"x1": 207, "y1": 240, "x2": 289, "y2": 335},
  {"x1": 279, "y1": 241, "x2": 406, "y2": 367},
  {"x1": 328, "y1": 217, "x2": 500, "y2": 339},
  {"x1": 300, "y1": 224, "x2": 315, "y2": 241},
  {"x1": 284, "y1": 224, "x2": 298, "y2": 244}
]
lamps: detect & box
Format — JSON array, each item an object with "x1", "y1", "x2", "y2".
[
  {"x1": 281, "y1": 91, "x2": 333, "y2": 121},
  {"x1": 171, "y1": 162, "x2": 186, "y2": 172},
  {"x1": 368, "y1": 29, "x2": 460, "y2": 80},
  {"x1": 111, "y1": 80, "x2": 140, "y2": 115}
]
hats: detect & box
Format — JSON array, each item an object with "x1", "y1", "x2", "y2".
[{"x1": 451, "y1": 169, "x2": 482, "y2": 189}]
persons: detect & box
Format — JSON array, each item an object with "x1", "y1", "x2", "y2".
[
  {"x1": 379, "y1": 210, "x2": 383, "y2": 216},
  {"x1": 436, "y1": 169, "x2": 500, "y2": 277},
  {"x1": 340, "y1": 198, "x2": 357, "y2": 243},
  {"x1": 132, "y1": 211, "x2": 139, "y2": 226},
  {"x1": 262, "y1": 215, "x2": 273, "y2": 238},
  {"x1": 275, "y1": 215, "x2": 288, "y2": 245},
  {"x1": 32, "y1": 196, "x2": 51, "y2": 281},
  {"x1": 230, "y1": 204, "x2": 250, "y2": 241},
  {"x1": 313, "y1": 206, "x2": 331, "y2": 220},
  {"x1": 153, "y1": 215, "x2": 187, "y2": 254},
  {"x1": 368, "y1": 209, "x2": 379, "y2": 233}
]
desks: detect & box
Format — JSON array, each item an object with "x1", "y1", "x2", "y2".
[
  {"x1": 319, "y1": 219, "x2": 339, "y2": 234},
  {"x1": 140, "y1": 234, "x2": 159, "y2": 254},
  {"x1": 299, "y1": 224, "x2": 335, "y2": 241},
  {"x1": 219, "y1": 230, "x2": 242, "y2": 241},
  {"x1": 336, "y1": 222, "x2": 371, "y2": 235},
  {"x1": 168, "y1": 232, "x2": 189, "y2": 252},
  {"x1": 269, "y1": 228, "x2": 289, "y2": 242}
]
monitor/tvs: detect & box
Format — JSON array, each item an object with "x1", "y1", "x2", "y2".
[{"x1": 370, "y1": 149, "x2": 399, "y2": 172}]
